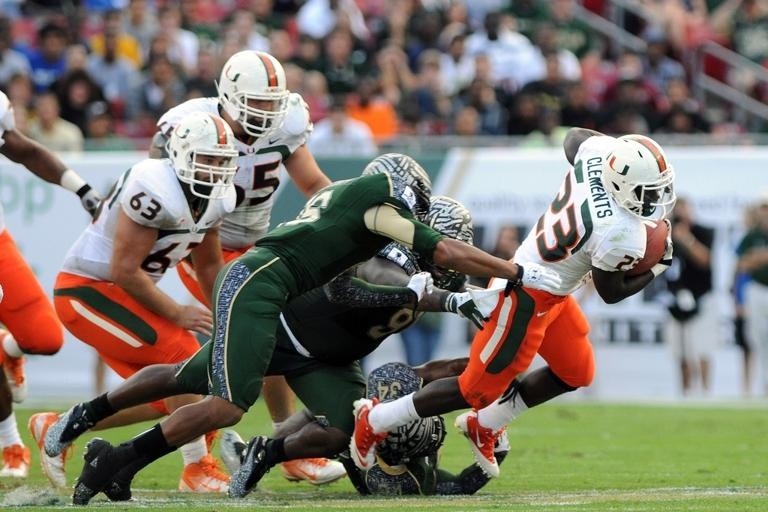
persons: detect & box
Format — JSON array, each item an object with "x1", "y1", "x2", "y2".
[
  {"x1": 29, "y1": 112, "x2": 236, "y2": 495},
  {"x1": 468, "y1": 226, "x2": 522, "y2": 345},
  {"x1": 348, "y1": 127, "x2": 675, "y2": 481},
  {"x1": 341, "y1": 357, "x2": 521, "y2": 496},
  {"x1": 73, "y1": 153, "x2": 563, "y2": 505},
  {"x1": 148, "y1": 51, "x2": 348, "y2": 485},
  {"x1": 398, "y1": 311, "x2": 443, "y2": 368},
  {"x1": 731, "y1": 202, "x2": 768, "y2": 397},
  {"x1": 662, "y1": 197, "x2": 716, "y2": 397},
  {"x1": 0, "y1": 91, "x2": 102, "y2": 478},
  {"x1": 44, "y1": 196, "x2": 492, "y2": 499},
  {"x1": 0, "y1": 0, "x2": 768, "y2": 145}
]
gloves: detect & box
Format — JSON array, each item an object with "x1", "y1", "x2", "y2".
[
  {"x1": 441, "y1": 291, "x2": 491, "y2": 330},
  {"x1": 514, "y1": 262, "x2": 561, "y2": 293},
  {"x1": 76, "y1": 183, "x2": 101, "y2": 223},
  {"x1": 407, "y1": 271, "x2": 434, "y2": 303},
  {"x1": 494, "y1": 428, "x2": 511, "y2": 453},
  {"x1": 650, "y1": 219, "x2": 673, "y2": 278}
]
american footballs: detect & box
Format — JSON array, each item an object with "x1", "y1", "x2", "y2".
[{"x1": 627, "y1": 221, "x2": 667, "y2": 276}]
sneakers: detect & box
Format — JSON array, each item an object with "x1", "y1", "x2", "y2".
[
  {"x1": 281, "y1": 457, "x2": 347, "y2": 486},
  {"x1": 0, "y1": 444, "x2": 30, "y2": 478},
  {"x1": 28, "y1": 413, "x2": 74, "y2": 489},
  {"x1": 221, "y1": 428, "x2": 249, "y2": 474},
  {"x1": 454, "y1": 409, "x2": 499, "y2": 479},
  {"x1": 103, "y1": 479, "x2": 132, "y2": 502},
  {"x1": 73, "y1": 438, "x2": 110, "y2": 507},
  {"x1": 228, "y1": 436, "x2": 271, "y2": 499},
  {"x1": 1, "y1": 330, "x2": 27, "y2": 404},
  {"x1": 349, "y1": 397, "x2": 389, "y2": 471},
  {"x1": 43, "y1": 402, "x2": 95, "y2": 459},
  {"x1": 179, "y1": 455, "x2": 231, "y2": 494}
]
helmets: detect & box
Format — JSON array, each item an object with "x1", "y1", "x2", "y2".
[
  {"x1": 601, "y1": 134, "x2": 676, "y2": 222},
  {"x1": 368, "y1": 363, "x2": 423, "y2": 405},
  {"x1": 165, "y1": 112, "x2": 238, "y2": 200},
  {"x1": 361, "y1": 152, "x2": 431, "y2": 213},
  {"x1": 414, "y1": 195, "x2": 473, "y2": 247},
  {"x1": 214, "y1": 50, "x2": 289, "y2": 137}
]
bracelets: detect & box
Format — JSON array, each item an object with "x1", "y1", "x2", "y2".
[{"x1": 60, "y1": 169, "x2": 86, "y2": 193}]
